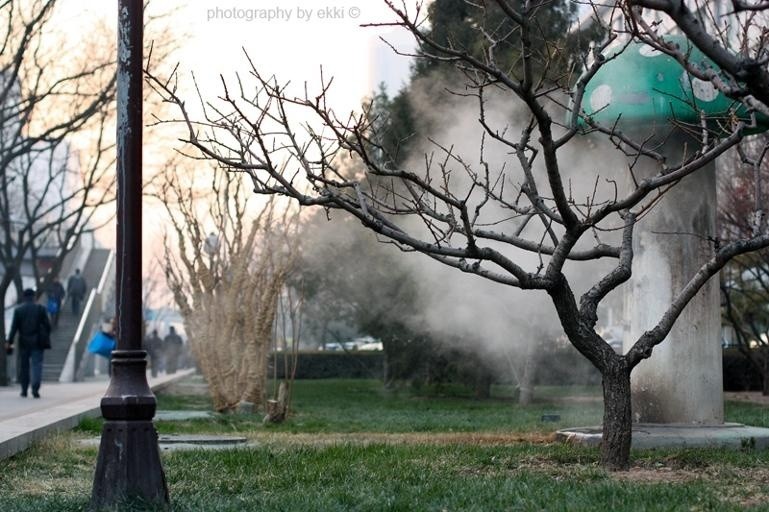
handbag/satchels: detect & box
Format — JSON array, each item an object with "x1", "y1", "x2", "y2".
[
  {"x1": 37, "y1": 304, "x2": 51, "y2": 349},
  {"x1": 48, "y1": 297, "x2": 59, "y2": 313},
  {"x1": 87, "y1": 330, "x2": 116, "y2": 360}
]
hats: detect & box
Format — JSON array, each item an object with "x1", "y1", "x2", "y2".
[{"x1": 22, "y1": 289, "x2": 35, "y2": 296}]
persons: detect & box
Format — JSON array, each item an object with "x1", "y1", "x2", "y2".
[
  {"x1": 46, "y1": 274, "x2": 65, "y2": 332},
  {"x1": 163, "y1": 325, "x2": 182, "y2": 375},
  {"x1": 67, "y1": 268, "x2": 87, "y2": 316},
  {"x1": 108, "y1": 317, "x2": 116, "y2": 335},
  {"x1": 145, "y1": 329, "x2": 166, "y2": 378},
  {"x1": 8, "y1": 289, "x2": 51, "y2": 402}
]
